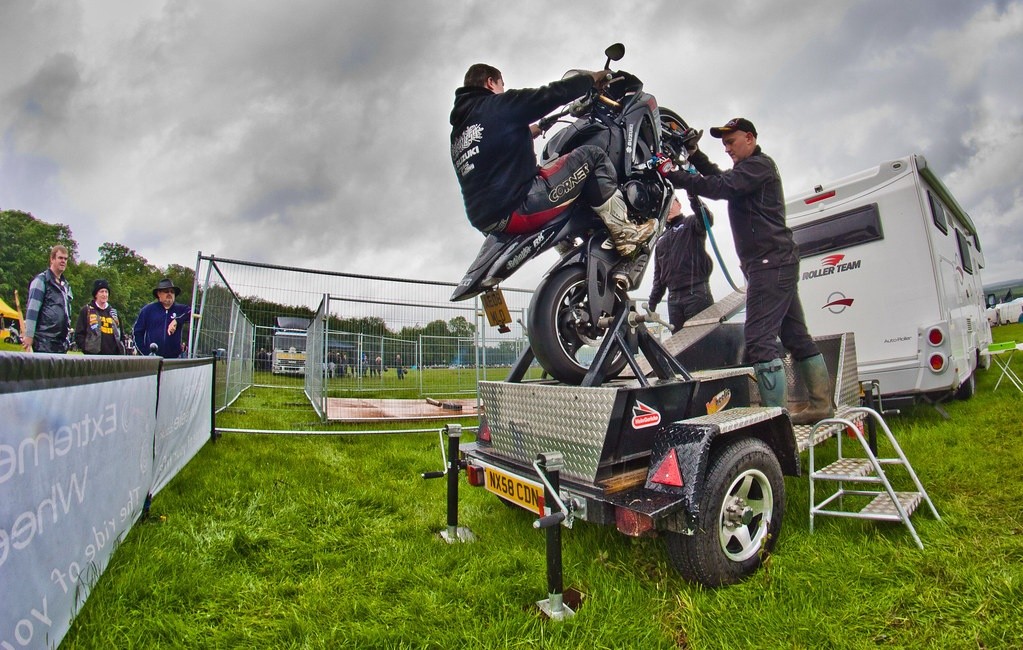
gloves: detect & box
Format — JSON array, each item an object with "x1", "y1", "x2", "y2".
[
  {"x1": 651, "y1": 152, "x2": 680, "y2": 178},
  {"x1": 680, "y1": 127, "x2": 704, "y2": 151}
]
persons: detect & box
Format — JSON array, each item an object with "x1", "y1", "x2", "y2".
[
  {"x1": 449, "y1": 63, "x2": 660, "y2": 257},
  {"x1": 211, "y1": 348, "x2": 405, "y2": 380},
  {"x1": 20, "y1": 245, "x2": 72, "y2": 354},
  {"x1": 131, "y1": 279, "x2": 200, "y2": 359},
  {"x1": 643, "y1": 190, "x2": 714, "y2": 335},
  {"x1": 75, "y1": 279, "x2": 125, "y2": 355},
  {"x1": 654, "y1": 117, "x2": 835, "y2": 425}
]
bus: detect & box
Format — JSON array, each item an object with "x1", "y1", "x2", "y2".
[{"x1": 781, "y1": 153, "x2": 996, "y2": 406}]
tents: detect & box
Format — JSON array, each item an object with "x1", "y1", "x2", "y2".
[{"x1": 0, "y1": 297, "x2": 22, "y2": 319}]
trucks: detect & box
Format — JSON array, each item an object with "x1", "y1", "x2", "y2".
[{"x1": 273, "y1": 330, "x2": 309, "y2": 377}]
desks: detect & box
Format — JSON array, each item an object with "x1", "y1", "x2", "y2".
[{"x1": 979, "y1": 344, "x2": 1023, "y2": 394}]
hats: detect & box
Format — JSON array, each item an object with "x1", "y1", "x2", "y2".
[
  {"x1": 92, "y1": 279, "x2": 110, "y2": 297},
  {"x1": 710, "y1": 118, "x2": 757, "y2": 138},
  {"x1": 153, "y1": 278, "x2": 181, "y2": 298}
]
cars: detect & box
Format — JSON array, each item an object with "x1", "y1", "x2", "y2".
[{"x1": 985, "y1": 285, "x2": 1023, "y2": 323}]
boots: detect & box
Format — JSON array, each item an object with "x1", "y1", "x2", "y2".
[
  {"x1": 753, "y1": 358, "x2": 789, "y2": 412},
  {"x1": 590, "y1": 187, "x2": 658, "y2": 258},
  {"x1": 788, "y1": 352, "x2": 836, "y2": 426}
]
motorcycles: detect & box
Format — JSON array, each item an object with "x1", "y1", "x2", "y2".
[
  {"x1": 3, "y1": 321, "x2": 23, "y2": 346},
  {"x1": 444, "y1": 41, "x2": 701, "y2": 388}
]
